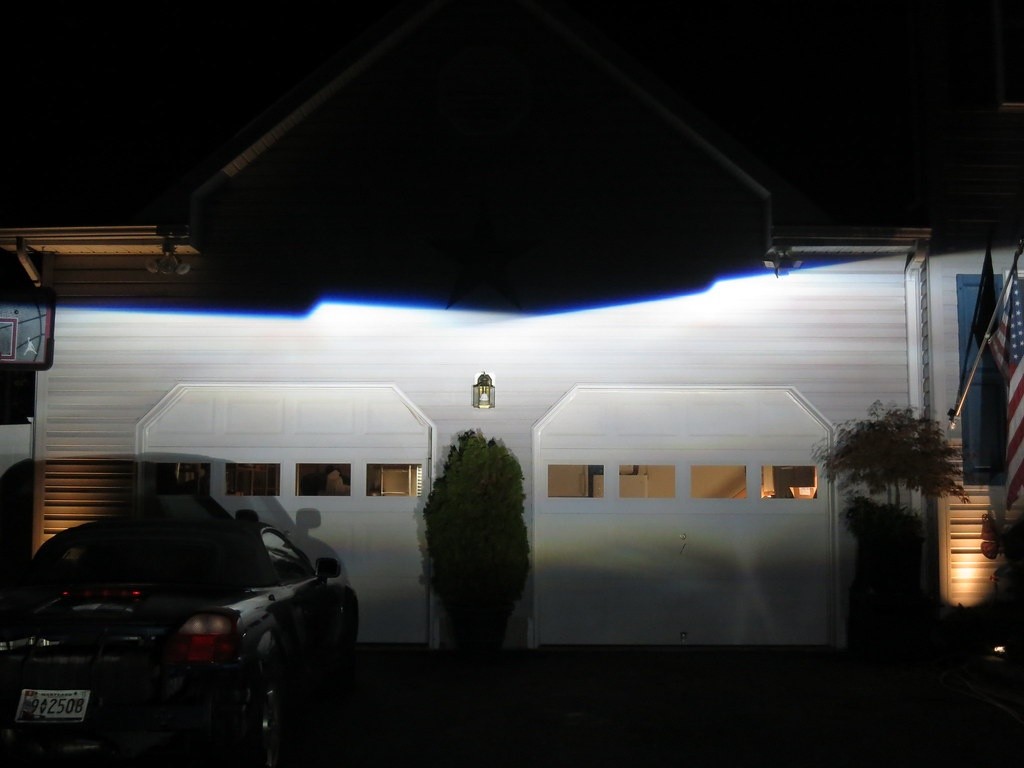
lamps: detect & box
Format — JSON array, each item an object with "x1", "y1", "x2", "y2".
[
  {"x1": 472, "y1": 375, "x2": 495, "y2": 410},
  {"x1": 763, "y1": 245, "x2": 803, "y2": 276},
  {"x1": 145, "y1": 239, "x2": 190, "y2": 276}
]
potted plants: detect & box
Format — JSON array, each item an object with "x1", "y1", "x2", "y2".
[{"x1": 422, "y1": 430, "x2": 533, "y2": 663}]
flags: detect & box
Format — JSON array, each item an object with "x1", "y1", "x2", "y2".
[{"x1": 988, "y1": 269, "x2": 1024, "y2": 535}]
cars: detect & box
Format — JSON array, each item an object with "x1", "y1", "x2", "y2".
[{"x1": 0, "y1": 516, "x2": 365, "y2": 768}]
